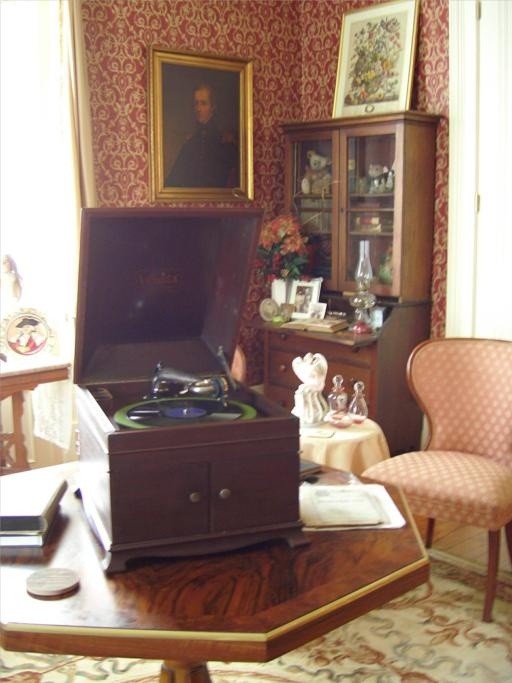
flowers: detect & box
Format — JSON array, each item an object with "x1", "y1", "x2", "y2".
[{"x1": 257, "y1": 216, "x2": 312, "y2": 279}]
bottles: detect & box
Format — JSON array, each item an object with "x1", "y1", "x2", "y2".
[
  {"x1": 327, "y1": 375, "x2": 348, "y2": 413},
  {"x1": 349, "y1": 380, "x2": 369, "y2": 423}
]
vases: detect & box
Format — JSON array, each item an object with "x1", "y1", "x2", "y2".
[{"x1": 270, "y1": 275, "x2": 287, "y2": 308}]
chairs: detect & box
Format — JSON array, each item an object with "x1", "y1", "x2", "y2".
[{"x1": 359, "y1": 338, "x2": 512, "y2": 622}]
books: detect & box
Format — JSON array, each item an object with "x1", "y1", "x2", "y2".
[
  {"x1": 281, "y1": 317, "x2": 349, "y2": 332},
  {"x1": 0, "y1": 472, "x2": 69, "y2": 546}
]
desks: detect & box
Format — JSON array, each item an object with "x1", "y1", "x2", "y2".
[
  {"x1": 1, "y1": 457, "x2": 431, "y2": 682},
  {"x1": 293, "y1": 412, "x2": 390, "y2": 474},
  {"x1": 0, "y1": 347, "x2": 72, "y2": 476}
]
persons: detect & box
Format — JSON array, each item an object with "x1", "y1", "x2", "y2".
[
  {"x1": 168, "y1": 85, "x2": 238, "y2": 188},
  {"x1": 295, "y1": 288, "x2": 311, "y2": 313},
  {"x1": 8, "y1": 318, "x2": 47, "y2": 353}
]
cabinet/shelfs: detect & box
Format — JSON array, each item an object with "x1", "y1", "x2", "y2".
[
  {"x1": 279, "y1": 111, "x2": 444, "y2": 303},
  {"x1": 257, "y1": 303, "x2": 431, "y2": 457}
]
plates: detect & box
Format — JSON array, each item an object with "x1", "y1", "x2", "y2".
[{"x1": 259, "y1": 298, "x2": 278, "y2": 321}]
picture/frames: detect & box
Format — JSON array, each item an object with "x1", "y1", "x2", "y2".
[
  {"x1": 331, "y1": 0, "x2": 419, "y2": 121},
  {"x1": 148, "y1": 44, "x2": 254, "y2": 203},
  {"x1": 291, "y1": 275, "x2": 324, "y2": 322}
]
